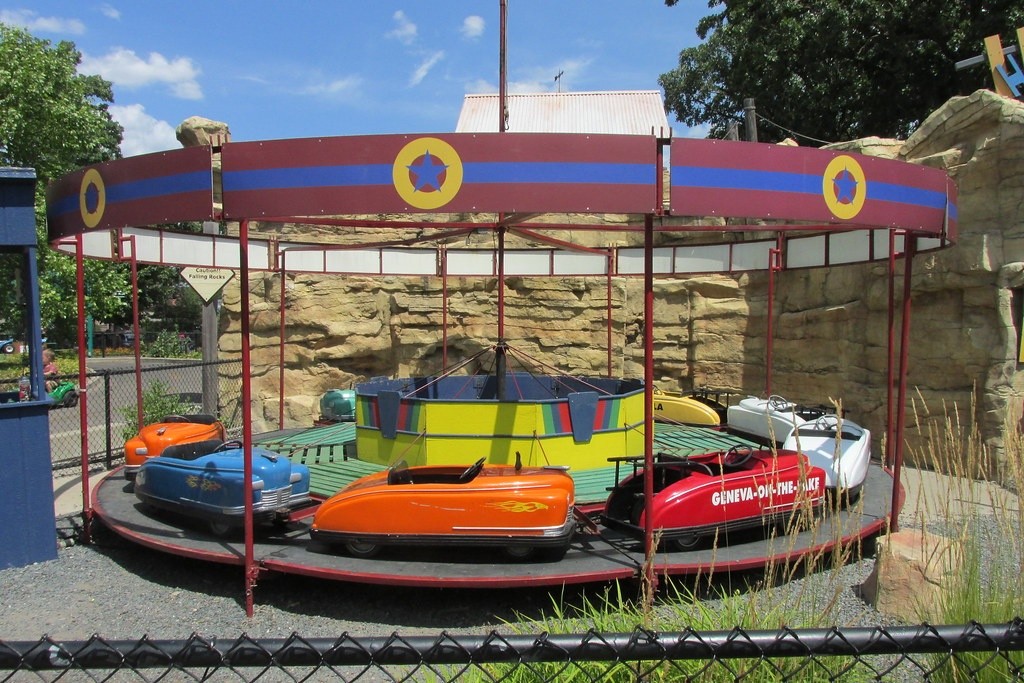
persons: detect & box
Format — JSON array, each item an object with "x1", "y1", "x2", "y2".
[{"x1": 42, "y1": 347, "x2": 60, "y2": 394}]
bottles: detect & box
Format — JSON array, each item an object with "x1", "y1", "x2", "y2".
[{"x1": 19, "y1": 377, "x2": 30, "y2": 402}]
